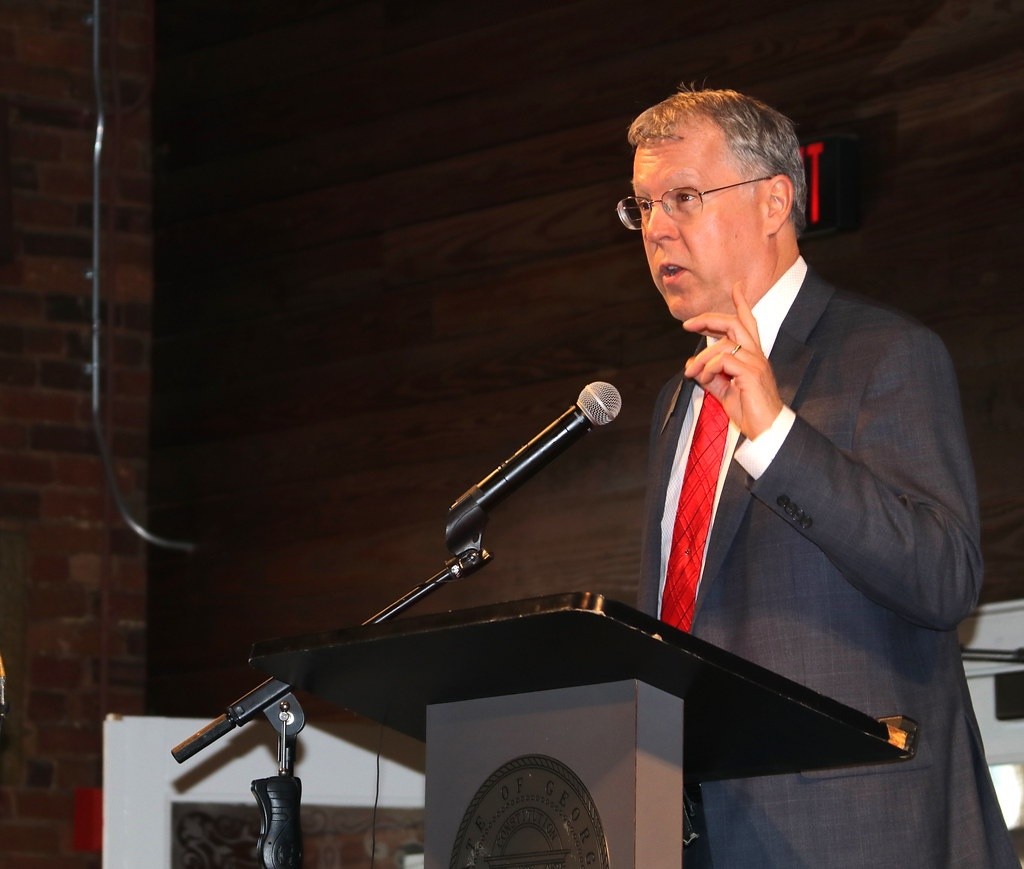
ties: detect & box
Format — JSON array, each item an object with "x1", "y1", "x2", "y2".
[{"x1": 660, "y1": 388, "x2": 730, "y2": 632}]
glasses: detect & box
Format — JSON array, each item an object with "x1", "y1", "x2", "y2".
[{"x1": 616, "y1": 176, "x2": 772, "y2": 229}]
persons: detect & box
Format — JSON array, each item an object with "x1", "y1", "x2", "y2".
[{"x1": 616, "y1": 90, "x2": 1022, "y2": 869}]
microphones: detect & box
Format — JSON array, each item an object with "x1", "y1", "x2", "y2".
[{"x1": 449, "y1": 381, "x2": 622, "y2": 518}]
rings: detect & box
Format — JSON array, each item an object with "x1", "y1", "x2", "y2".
[{"x1": 732, "y1": 344, "x2": 741, "y2": 356}]
